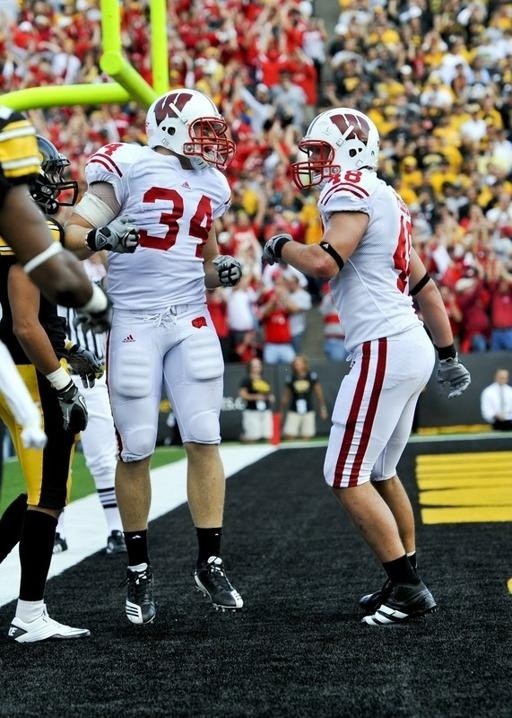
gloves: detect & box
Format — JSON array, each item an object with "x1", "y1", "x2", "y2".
[
  {"x1": 61, "y1": 340, "x2": 103, "y2": 388},
  {"x1": 436, "y1": 351, "x2": 471, "y2": 399},
  {"x1": 261, "y1": 234, "x2": 293, "y2": 266},
  {"x1": 86, "y1": 215, "x2": 138, "y2": 254},
  {"x1": 211, "y1": 254, "x2": 241, "y2": 287},
  {"x1": 72, "y1": 281, "x2": 114, "y2": 334},
  {"x1": 56, "y1": 379, "x2": 87, "y2": 435}
]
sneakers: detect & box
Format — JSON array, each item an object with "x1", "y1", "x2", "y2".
[
  {"x1": 362, "y1": 576, "x2": 437, "y2": 626},
  {"x1": 8, "y1": 603, "x2": 90, "y2": 644},
  {"x1": 192, "y1": 555, "x2": 244, "y2": 614},
  {"x1": 107, "y1": 530, "x2": 127, "y2": 554},
  {"x1": 51, "y1": 532, "x2": 67, "y2": 554},
  {"x1": 125, "y1": 562, "x2": 155, "y2": 626},
  {"x1": 358, "y1": 567, "x2": 417, "y2": 613}
]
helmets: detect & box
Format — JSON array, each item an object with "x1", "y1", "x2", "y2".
[
  {"x1": 290, "y1": 107, "x2": 380, "y2": 190},
  {"x1": 145, "y1": 88, "x2": 236, "y2": 171},
  {"x1": 28, "y1": 136, "x2": 78, "y2": 214}
]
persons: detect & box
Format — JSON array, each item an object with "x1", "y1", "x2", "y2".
[
  {"x1": 61, "y1": 89, "x2": 243, "y2": 625},
  {"x1": 1, "y1": 13, "x2": 111, "y2": 643},
  {"x1": 1, "y1": 0, "x2": 512, "y2": 443},
  {"x1": 262, "y1": 108, "x2": 469, "y2": 625}
]
